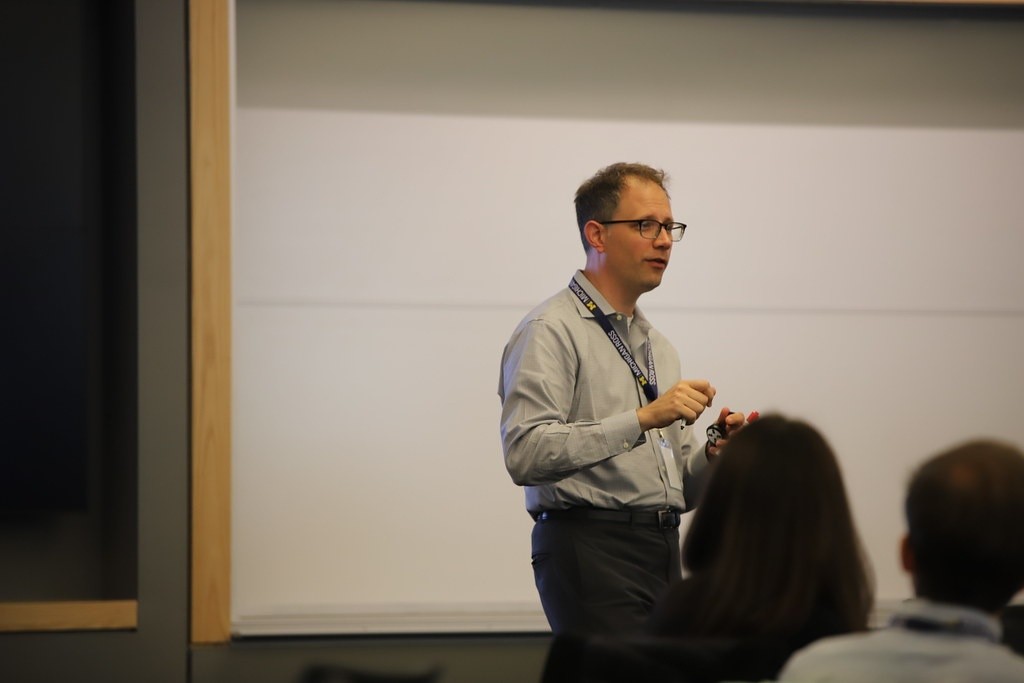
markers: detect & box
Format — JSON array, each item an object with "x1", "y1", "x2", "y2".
[{"x1": 680, "y1": 419, "x2": 686, "y2": 430}]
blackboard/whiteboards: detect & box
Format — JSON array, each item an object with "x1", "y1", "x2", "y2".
[
  {"x1": 230, "y1": 0, "x2": 1024, "y2": 639},
  {"x1": 0, "y1": 1, "x2": 139, "y2": 629}
]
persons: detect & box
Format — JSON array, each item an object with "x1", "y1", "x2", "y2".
[
  {"x1": 499, "y1": 163, "x2": 746, "y2": 682},
  {"x1": 780, "y1": 441, "x2": 1024, "y2": 683},
  {"x1": 539, "y1": 412, "x2": 875, "y2": 682}
]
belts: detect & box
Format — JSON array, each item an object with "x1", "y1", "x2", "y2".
[{"x1": 539, "y1": 507, "x2": 682, "y2": 529}]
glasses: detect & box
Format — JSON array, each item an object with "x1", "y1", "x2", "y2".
[{"x1": 601, "y1": 219, "x2": 688, "y2": 242}]
